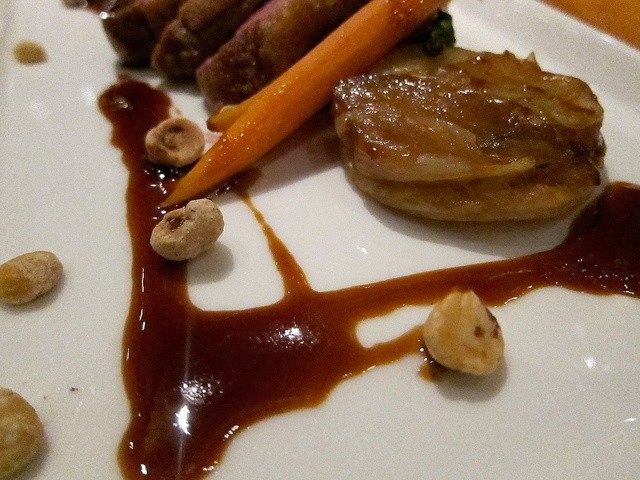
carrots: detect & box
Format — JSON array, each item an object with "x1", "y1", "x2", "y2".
[
  {"x1": 156, "y1": 0, "x2": 451, "y2": 210},
  {"x1": 204, "y1": 86, "x2": 265, "y2": 134}
]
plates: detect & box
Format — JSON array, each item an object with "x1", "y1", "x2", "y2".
[{"x1": 0, "y1": 2, "x2": 640, "y2": 479}]
